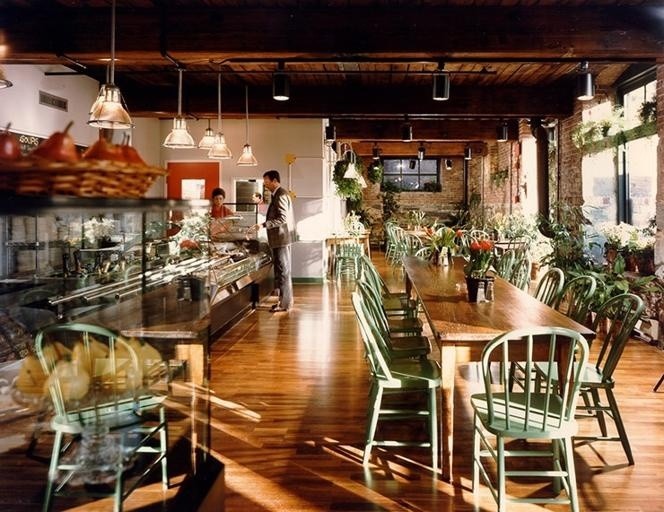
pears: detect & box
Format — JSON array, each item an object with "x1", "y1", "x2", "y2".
[
  {"x1": 18, "y1": 332, "x2": 163, "y2": 402},
  {"x1": 0, "y1": 121, "x2": 145, "y2": 165}
]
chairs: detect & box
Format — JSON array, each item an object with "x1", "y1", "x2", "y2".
[
  {"x1": 493, "y1": 245, "x2": 646, "y2": 466},
  {"x1": 468, "y1": 324, "x2": 592, "y2": 512},
  {"x1": 33, "y1": 323, "x2": 169, "y2": 511},
  {"x1": 382, "y1": 219, "x2": 537, "y2": 269},
  {"x1": 325, "y1": 218, "x2": 372, "y2": 283},
  {"x1": 347, "y1": 250, "x2": 442, "y2": 478}
]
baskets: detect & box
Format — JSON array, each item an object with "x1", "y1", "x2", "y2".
[{"x1": 0, "y1": 158, "x2": 168, "y2": 198}]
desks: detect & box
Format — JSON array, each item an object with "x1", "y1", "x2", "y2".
[{"x1": 396, "y1": 251, "x2": 597, "y2": 482}]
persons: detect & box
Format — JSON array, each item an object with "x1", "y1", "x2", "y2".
[
  {"x1": 252, "y1": 193, "x2": 267, "y2": 212},
  {"x1": 254, "y1": 170, "x2": 296, "y2": 311},
  {"x1": 211, "y1": 187, "x2": 232, "y2": 216}
]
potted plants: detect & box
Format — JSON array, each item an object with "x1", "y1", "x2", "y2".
[{"x1": 570, "y1": 95, "x2": 656, "y2": 150}]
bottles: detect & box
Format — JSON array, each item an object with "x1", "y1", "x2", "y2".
[
  {"x1": 475, "y1": 281, "x2": 494, "y2": 304},
  {"x1": 435, "y1": 252, "x2": 449, "y2": 267}
]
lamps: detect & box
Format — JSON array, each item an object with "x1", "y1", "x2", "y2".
[
  {"x1": 87, "y1": 1, "x2": 259, "y2": 169},
  {"x1": 574, "y1": 60, "x2": 597, "y2": 101},
  {"x1": 324, "y1": 116, "x2": 510, "y2": 174},
  {"x1": 266, "y1": 69, "x2": 496, "y2": 105}
]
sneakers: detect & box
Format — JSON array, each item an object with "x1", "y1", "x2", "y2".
[{"x1": 269, "y1": 303, "x2": 287, "y2": 312}]
